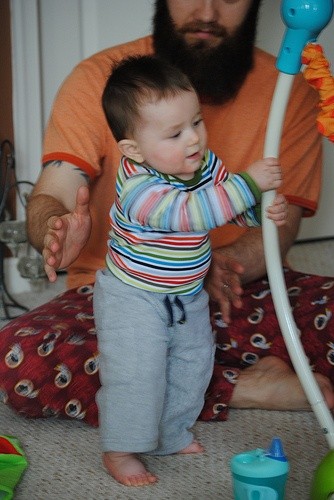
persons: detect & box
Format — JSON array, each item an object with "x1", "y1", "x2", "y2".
[
  {"x1": 94, "y1": 54, "x2": 290, "y2": 487},
  {"x1": 0, "y1": 0, "x2": 333, "y2": 423}
]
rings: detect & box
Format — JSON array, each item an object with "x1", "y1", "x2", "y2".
[{"x1": 222, "y1": 283, "x2": 229, "y2": 295}]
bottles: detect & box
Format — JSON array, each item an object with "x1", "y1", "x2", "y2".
[{"x1": 230, "y1": 439, "x2": 288, "y2": 500}]
